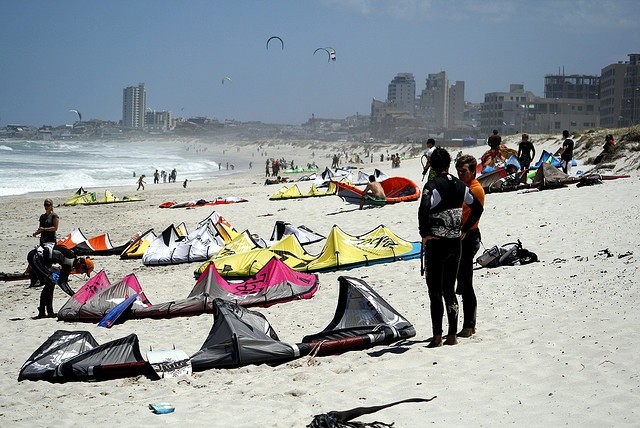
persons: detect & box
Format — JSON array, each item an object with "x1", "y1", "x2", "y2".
[
  {"x1": 360, "y1": 177, "x2": 388, "y2": 210},
  {"x1": 135, "y1": 174, "x2": 146, "y2": 191},
  {"x1": 27, "y1": 245, "x2": 94, "y2": 319},
  {"x1": 488, "y1": 130, "x2": 503, "y2": 151},
  {"x1": 34, "y1": 198, "x2": 60, "y2": 242},
  {"x1": 516, "y1": 133, "x2": 536, "y2": 174},
  {"x1": 422, "y1": 139, "x2": 438, "y2": 180},
  {"x1": 418, "y1": 148, "x2": 484, "y2": 348},
  {"x1": 593, "y1": 133, "x2": 614, "y2": 164},
  {"x1": 557, "y1": 130, "x2": 575, "y2": 175},
  {"x1": 456, "y1": 155, "x2": 487, "y2": 338},
  {"x1": 154, "y1": 131, "x2": 417, "y2": 189}
]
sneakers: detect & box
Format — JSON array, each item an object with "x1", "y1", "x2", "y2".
[
  {"x1": 457, "y1": 328, "x2": 472, "y2": 337},
  {"x1": 427, "y1": 336, "x2": 442, "y2": 347},
  {"x1": 443, "y1": 335, "x2": 458, "y2": 345}
]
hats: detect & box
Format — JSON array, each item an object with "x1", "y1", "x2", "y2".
[{"x1": 85, "y1": 257, "x2": 94, "y2": 277}]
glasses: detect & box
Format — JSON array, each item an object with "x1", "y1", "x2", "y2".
[{"x1": 44, "y1": 203, "x2": 51, "y2": 206}]
